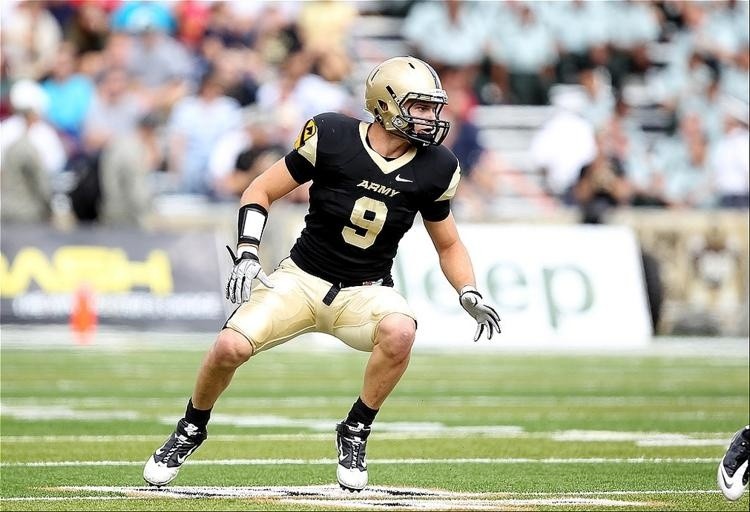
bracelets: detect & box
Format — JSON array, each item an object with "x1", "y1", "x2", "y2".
[{"x1": 233, "y1": 203, "x2": 268, "y2": 249}]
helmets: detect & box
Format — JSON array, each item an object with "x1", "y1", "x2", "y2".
[{"x1": 365, "y1": 56, "x2": 450, "y2": 147}]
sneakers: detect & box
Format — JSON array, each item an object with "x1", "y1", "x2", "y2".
[
  {"x1": 717, "y1": 424, "x2": 750, "y2": 501},
  {"x1": 334, "y1": 422, "x2": 371, "y2": 492},
  {"x1": 141, "y1": 419, "x2": 208, "y2": 488}
]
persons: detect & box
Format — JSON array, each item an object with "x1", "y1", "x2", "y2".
[
  {"x1": 0, "y1": 2, "x2": 750, "y2": 235},
  {"x1": 712, "y1": 423, "x2": 750, "y2": 504},
  {"x1": 137, "y1": 54, "x2": 503, "y2": 496}
]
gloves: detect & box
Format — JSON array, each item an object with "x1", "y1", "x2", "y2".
[
  {"x1": 459, "y1": 285, "x2": 501, "y2": 342},
  {"x1": 224, "y1": 246, "x2": 275, "y2": 305}
]
